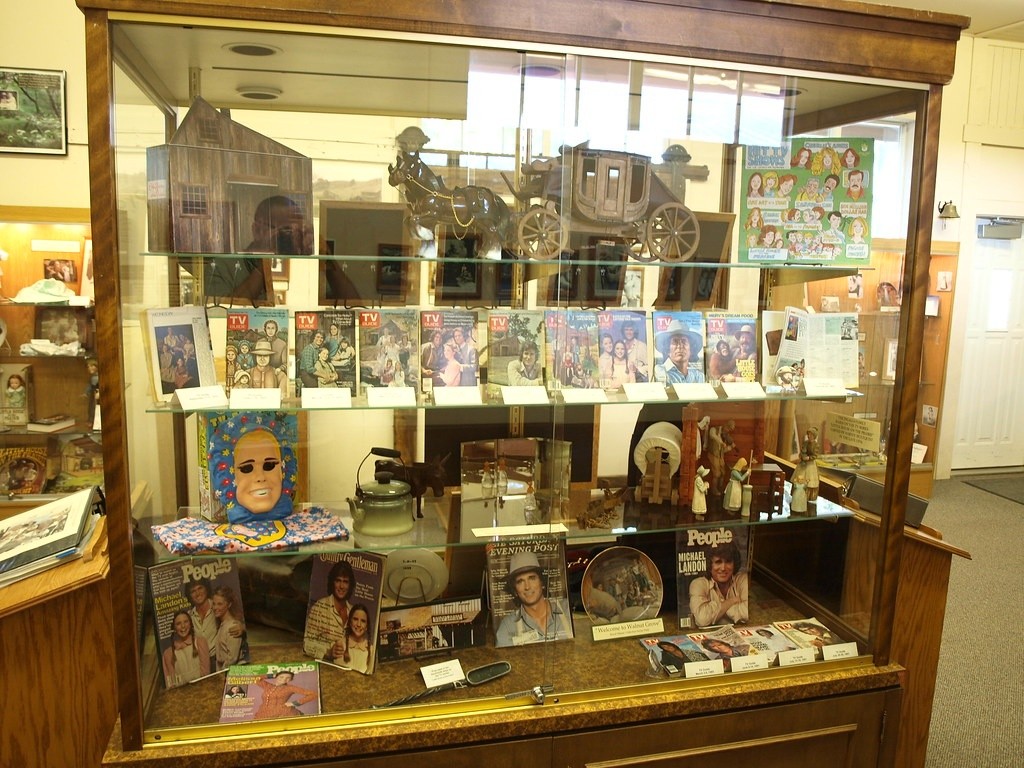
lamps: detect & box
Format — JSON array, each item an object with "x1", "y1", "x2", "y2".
[{"x1": 938, "y1": 200, "x2": 960, "y2": 220}]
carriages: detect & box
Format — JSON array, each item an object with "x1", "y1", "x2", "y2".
[{"x1": 386, "y1": 125, "x2": 710, "y2": 265}]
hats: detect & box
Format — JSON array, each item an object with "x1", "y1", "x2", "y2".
[
  {"x1": 734, "y1": 325, "x2": 755, "y2": 347},
  {"x1": 500, "y1": 552, "x2": 546, "y2": 582},
  {"x1": 655, "y1": 320, "x2": 703, "y2": 358}
]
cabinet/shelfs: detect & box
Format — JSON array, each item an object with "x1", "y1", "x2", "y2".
[{"x1": 77, "y1": 2, "x2": 970, "y2": 768}]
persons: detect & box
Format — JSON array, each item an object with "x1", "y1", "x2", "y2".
[
  {"x1": 926, "y1": 407, "x2": 935, "y2": 425},
  {"x1": 654, "y1": 320, "x2": 705, "y2": 383},
  {"x1": 709, "y1": 340, "x2": 736, "y2": 382},
  {"x1": 421, "y1": 327, "x2": 477, "y2": 387},
  {"x1": 208, "y1": 196, "x2": 363, "y2": 305},
  {"x1": 46, "y1": 260, "x2": 71, "y2": 280},
  {"x1": 162, "y1": 551, "x2": 572, "y2": 723},
  {"x1": 4, "y1": 375, "x2": 26, "y2": 407},
  {"x1": 374, "y1": 327, "x2": 411, "y2": 388},
  {"x1": 657, "y1": 540, "x2": 846, "y2": 677},
  {"x1": 692, "y1": 419, "x2": 819, "y2": 521},
  {"x1": 599, "y1": 319, "x2": 648, "y2": 388},
  {"x1": 732, "y1": 325, "x2": 757, "y2": 360},
  {"x1": 552, "y1": 334, "x2": 593, "y2": 387},
  {"x1": 508, "y1": 341, "x2": 541, "y2": 386}
]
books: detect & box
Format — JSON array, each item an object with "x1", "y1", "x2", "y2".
[
  {"x1": 674, "y1": 523, "x2": 749, "y2": 631},
  {"x1": 149, "y1": 534, "x2": 573, "y2": 690},
  {"x1": 139, "y1": 305, "x2": 217, "y2": 407},
  {"x1": 640, "y1": 616, "x2": 849, "y2": 678},
  {"x1": 543, "y1": 309, "x2": 600, "y2": 391},
  {"x1": 597, "y1": 311, "x2": 649, "y2": 389},
  {"x1": 218, "y1": 658, "x2": 321, "y2": 723},
  {"x1": 226, "y1": 308, "x2": 289, "y2": 398},
  {"x1": 295, "y1": 310, "x2": 356, "y2": 398},
  {"x1": 487, "y1": 309, "x2": 546, "y2": 399},
  {"x1": 27, "y1": 414, "x2": 76, "y2": 433},
  {"x1": 0, "y1": 483, "x2": 101, "y2": 590},
  {"x1": 358, "y1": 307, "x2": 419, "y2": 394},
  {"x1": 652, "y1": 306, "x2": 859, "y2": 394},
  {"x1": 421, "y1": 311, "x2": 479, "y2": 392}
]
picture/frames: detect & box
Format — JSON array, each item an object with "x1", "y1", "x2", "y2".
[{"x1": 0, "y1": 67, "x2": 68, "y2": 155}]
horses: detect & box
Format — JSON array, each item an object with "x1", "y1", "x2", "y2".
[{"x1": 388, "y1": 150, "x2": 508, "y2": 260}]
